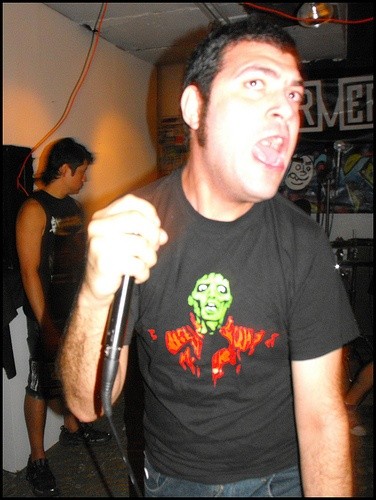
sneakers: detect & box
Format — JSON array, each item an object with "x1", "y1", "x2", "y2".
[
  {"x1": 343, "y1": 401, "x2": 367, "y2": 436},
  {"x1": 26, "y1": 453, "x2": 61, "y2": 498},
  {"x1": 60, "y1": 421, "x2": 112, "y2": 445}
]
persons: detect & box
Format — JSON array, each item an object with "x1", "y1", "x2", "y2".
[
  {"x1": 17, "y1": 136, "x2": 110, "y2": 498},
  {"x1": 342, "y1": 337, "x2": 373, "y2": 407},
  {"x1": 60, "y1": 19, "x2": 360, "y2": 497}
]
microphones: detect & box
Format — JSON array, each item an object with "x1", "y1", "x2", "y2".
[
  {"x1": 100, "y1": 275, "x2": 136, "y2": 416},
  {"x1": 333, "y1": 140, "x2": 345, "y2": 191}
]
